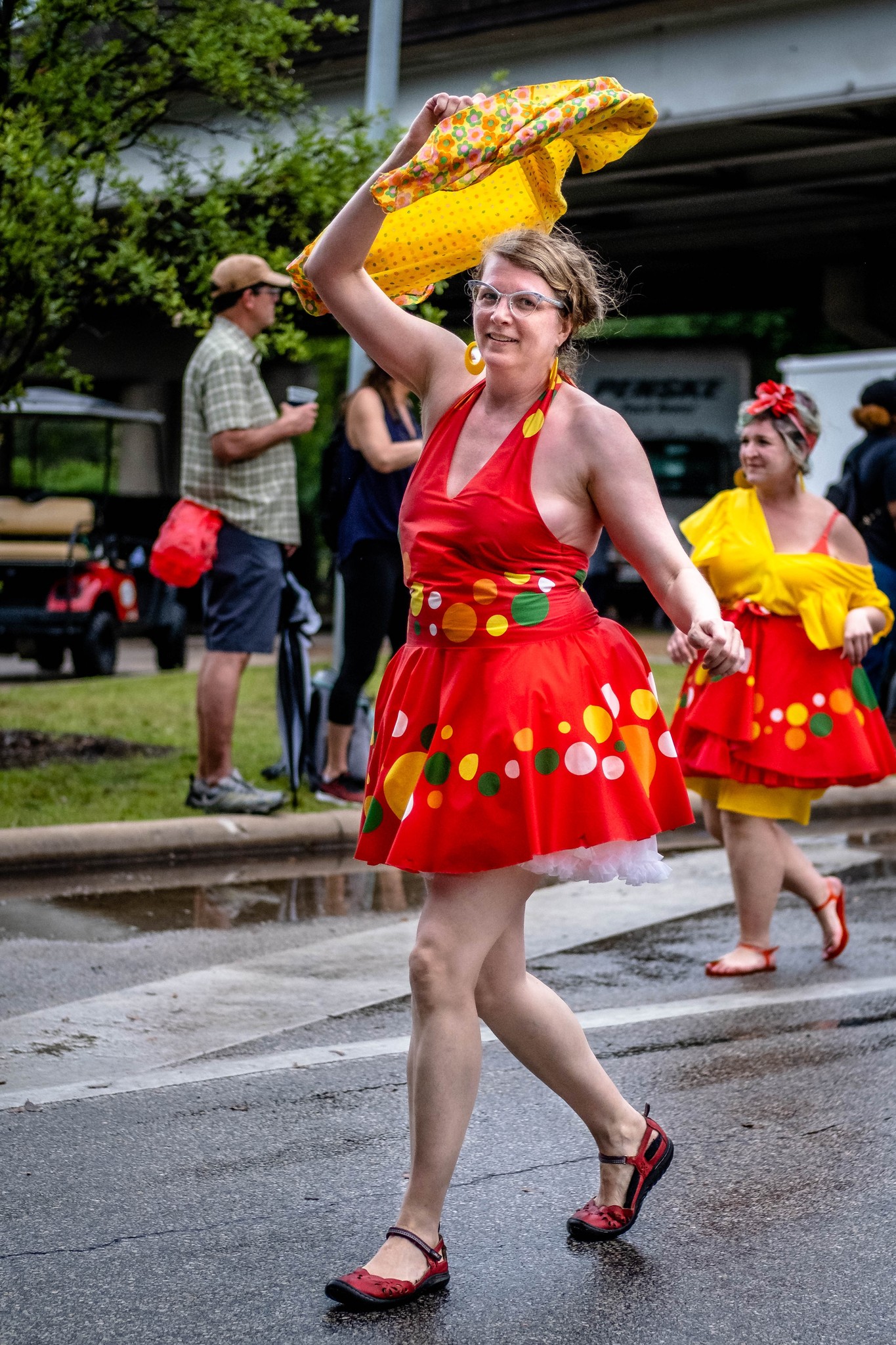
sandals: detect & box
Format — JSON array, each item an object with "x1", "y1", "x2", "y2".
[
  {"x1": 702, "y1": 943, "x2": 779, "y2": 978},
  {"x1": 810, "y1": 875, "x2": 849, "y2": 962}
]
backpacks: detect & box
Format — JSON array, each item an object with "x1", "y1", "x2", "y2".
[
  {"x1": 824, "y1": 432, "x2": 896, "y2": 573},
  {"x1": 316, "y1": 384, "x2": 393, "y2": 563}
]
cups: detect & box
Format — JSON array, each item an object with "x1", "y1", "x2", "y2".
[{"x1": 286, "y1": 384, "x2": 319, "y2": 408}]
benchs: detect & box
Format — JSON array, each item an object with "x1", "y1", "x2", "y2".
[{"x1": 0, "y1": 496, "x2": 98, "y2": 568}]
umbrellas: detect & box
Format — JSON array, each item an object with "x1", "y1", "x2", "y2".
[{"x1": 277, "y1": 545, "x2": 323, "y2": 807}]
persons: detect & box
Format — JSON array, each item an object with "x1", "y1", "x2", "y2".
[
  {"x1": 183, "y1": 253, "x2": 424, "y2": 816},
  {"x1": 668, "y1": 381, "x2": 894, "y2": 974},
  {"x1": 823, "y1": 377, "x2": 896, "y2": 731},
  {"x1": 302, "y1": 91, "x2": 746, "y2": 1303}
]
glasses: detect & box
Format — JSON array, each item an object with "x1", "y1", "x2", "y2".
[
  {"x1": 252, "y1": 286, "x2": 282, "y2": 298},
  {"x1": 468, "y1": 279, "x2": 565, "y2": 319}
]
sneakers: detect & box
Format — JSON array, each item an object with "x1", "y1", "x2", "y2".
[
  {"x1": 313, "y1": 771, "x2": 366, "y2": 810},
  {"x1": 184, "y1": 765, "x2": 286, "y2": 816}
]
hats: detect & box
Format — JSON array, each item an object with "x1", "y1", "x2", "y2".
[{"x1": 206, "y1": 253, "x2": 293, "y2": 302}]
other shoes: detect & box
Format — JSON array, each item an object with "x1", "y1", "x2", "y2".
[
  {"x1": 322, "y1": 1223, "x2": 452, "y2": 1312},
  {"x1": 565, "y1": 1102, "x2": 674, "y2": 1242}
]
